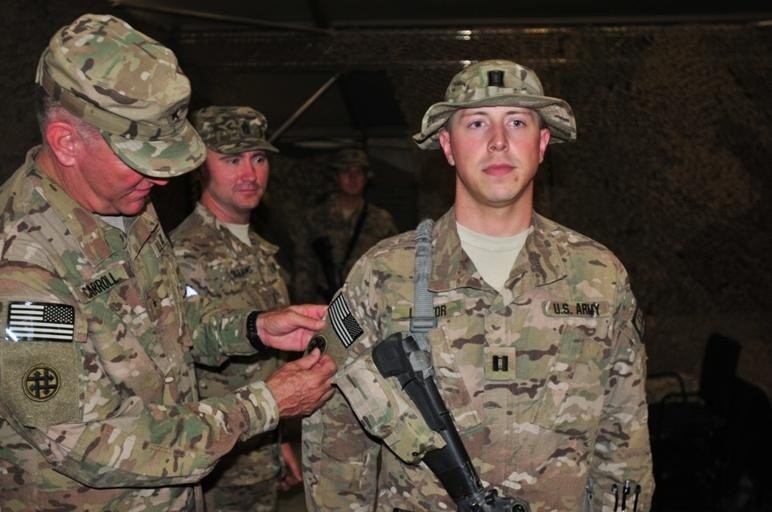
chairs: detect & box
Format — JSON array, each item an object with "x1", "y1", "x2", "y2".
[{"x1": 644, "y1": 331, "x2": 771, "y2": 512}]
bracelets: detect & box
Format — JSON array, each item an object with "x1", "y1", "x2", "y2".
[{"x1": 246, "y1": 310, "x2": 267, "y2": 354}]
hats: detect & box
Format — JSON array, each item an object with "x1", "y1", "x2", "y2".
[
  {"x1": 34, "y1": 12, "x2": 208, "y2": 179},
  {"x1": 412, "y1": 58, "x2": 578, "y2": 152},
  {"x1": 331, "y1": 149, "x2": 372, "y2": 172},
  {"x1": 193, "y1": 104, "x2": 280, "y2": 156}
]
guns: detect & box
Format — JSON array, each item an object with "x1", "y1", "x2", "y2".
[{"x1": 371, "y1": 333, "x2": 531, "y2": 512}]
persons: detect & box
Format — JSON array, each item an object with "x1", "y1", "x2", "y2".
[
  {"x1": 0, "y1": 15, "x2": 341, "y2": 511},
  {"x1": 302, "y1": 60, "x2": 656, "y2": 511},
  {"x1": 163, "y1": 104, "x2": 305, "y2": 510},
  {"x1": 293, "y1": 138, "x2": 399, "y2": 303}
]
